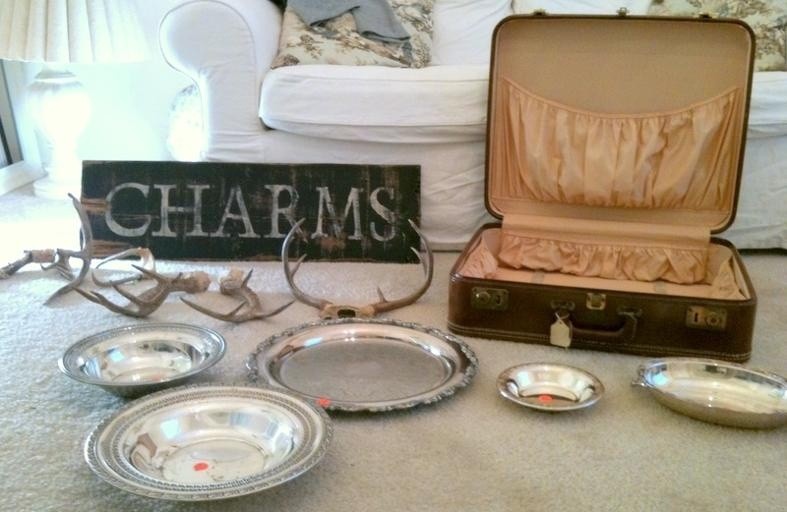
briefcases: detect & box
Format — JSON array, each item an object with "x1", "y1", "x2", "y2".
[{"x1": 445, "y1": 2, "x2": 758, "y2": 364}]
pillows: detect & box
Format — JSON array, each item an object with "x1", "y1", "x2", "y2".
[
  {"x1": 270, "y1": 2, "x2": 436, "y2": 68},
  {"x1": 641, "y1": 0, "x2": 783, "y2": 69}
]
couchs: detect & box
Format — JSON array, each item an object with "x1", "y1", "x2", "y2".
[{"x1": 157, "y1": 0, "x2": 787, "y2": 261}]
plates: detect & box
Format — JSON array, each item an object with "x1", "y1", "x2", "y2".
[
  {"x1": 496, "y1": 362, "x2": 606, "y2": 414},
  {"x1": 630, "y1": 357, "x2": 787, "y2": 429},
  {"x1": 83, "y1": 381, "x2": 334, "y2": 503},
  {"x1": 244, "y1": 315, "x2": 477, "y2": 416}
]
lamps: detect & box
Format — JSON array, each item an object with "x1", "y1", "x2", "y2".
[{"x1": 0, "y1": 0, "x2": 151, "y2": 204}]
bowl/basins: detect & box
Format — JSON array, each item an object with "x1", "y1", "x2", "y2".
[{"x1": 55, "y1": 321, "x2": 226, "y2": 399}]
